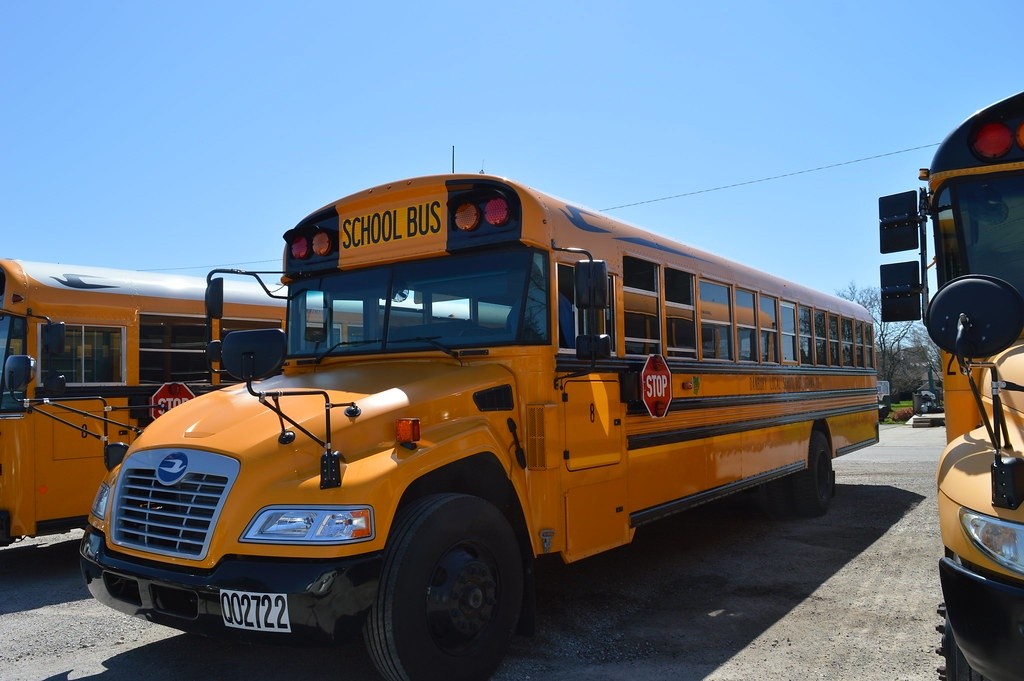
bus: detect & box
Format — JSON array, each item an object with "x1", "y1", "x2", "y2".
[
  {"x1": 2, "y1": 172, "x2": 882, "y2": 681},
  {"x1": 1, "y1": 257, "x2": 512, "y2": 548},
  {"x1": 877, "y1": 93, "x2": 1024, "y2": 681}
]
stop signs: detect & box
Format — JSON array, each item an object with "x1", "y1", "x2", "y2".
[
  {"x1": 638, "y1": 353, "x2": 673, "y2": 418},
  {"x1": 150, "y1": 380, "x2": 196, "y2": 421}
]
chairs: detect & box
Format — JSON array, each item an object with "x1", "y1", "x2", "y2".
[{"x1": 506, "y1": 290, "x2": 574, "y2": 347}]
